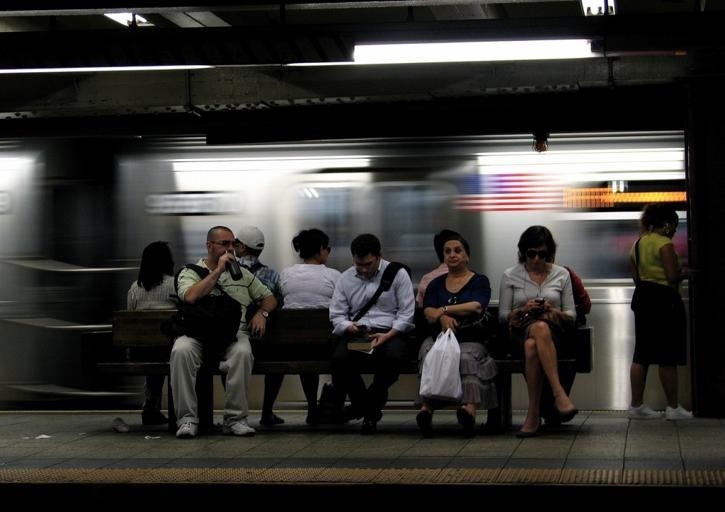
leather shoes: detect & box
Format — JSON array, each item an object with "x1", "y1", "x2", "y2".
[
  {"x1": 517, "y1": 418, "x2": 541, "y2": 438},
  {"x1": 553, "y1": 404, "x2": 578, "y2": 422},
  {"x1": 306, "y1": 406, "x2": 383, "y2": 433}
]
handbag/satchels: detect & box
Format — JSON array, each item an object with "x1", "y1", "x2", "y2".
[
  {"x1": 577, "y1": 327, "x2": 593, "y2": 373},
  {"x1": 508, "y1": 309, "x2": 568, "y2": 333},
  {"x1": 318, "y1": 331, "x2": 343, "y2": 360},
  {"x1": 631, "y1": 281, "x2": 673, "y2": 313},
  {"x1": 176, "y1": 293, "x2": 241, "y2": 347},
  {"x1": 430, "y1": 299, "x2": 488, "y2": 340}
]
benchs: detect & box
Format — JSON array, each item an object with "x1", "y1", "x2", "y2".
[{"x1": 94, "y1": 306, "x2": 593, "y2": 437}]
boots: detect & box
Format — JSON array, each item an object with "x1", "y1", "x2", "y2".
[{"x1": 142, "y1": 384, "x2": 167, "y2": 424}]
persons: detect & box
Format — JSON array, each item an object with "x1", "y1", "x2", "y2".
[
  {"x1": 498, "y1": 225, "x2": 579, "y2": 437},
  {"x1": 126, "y1": 241, "x2": 176, "y2": 425},
  {"x1": 329, "y1": 233, "x2": 415, "y2": 430},
  {"x1": 521, "y1": 265, "x2": 591, "y2": 423},
  {"x1": 628, "y1": 201, "x2": 694, "y2": 419},
  {"x1": 221, "y1": 231, "x2": 285, "y2": 425},
  {"x1": 169, "y1": 226, "x2": 279, "y2": 438},
  {"x1": 416, "y1": 235, "x2": 491, "y2": 438},
  {"x1": 280, "y1": 228, "x2": 348, "y2": 427},
  {"x1": 416, "y1": 229, "x2": 460, "y2": 307}
]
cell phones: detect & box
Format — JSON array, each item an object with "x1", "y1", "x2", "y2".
[
  {"x1": 535, "y1": 298, "x2": 544, "y2": 306},
  {"x1": 356, "y1": 324, "x2": 367, "y2": 328}
]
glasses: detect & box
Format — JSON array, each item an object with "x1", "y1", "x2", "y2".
[
  {"x1": 211, "y1": 240, "x2": 235, "y2": 246},
  {"x1": 526, "y1": 250, "x2": 547, "y2": 258},
  {"x1": 322, "y1": 245, "x2": 330, "y2": 253}
]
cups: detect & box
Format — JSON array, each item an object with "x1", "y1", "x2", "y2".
[{"x1": 223, "y1": 250, "x2": 243, "y2": 281}]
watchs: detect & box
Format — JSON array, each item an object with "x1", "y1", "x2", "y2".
[
  {"x1": 441, "y1": 304, "x2": 449, "y2": 313},
  {"x1": 259, "y1": 310, "x2": 269, "y2": 317}
]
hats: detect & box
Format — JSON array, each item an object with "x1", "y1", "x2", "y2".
[{"x1": 234, "y1": 230, "x2": 264, "y2": 250}]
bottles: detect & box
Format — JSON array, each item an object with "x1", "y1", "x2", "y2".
[{"x1": 111, "y1": 418, "x2": 130, "y2": 432}]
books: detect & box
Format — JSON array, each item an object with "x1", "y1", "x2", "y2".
[{"x1": 348, "y1": 336, "x2": 378, "y2": 351}]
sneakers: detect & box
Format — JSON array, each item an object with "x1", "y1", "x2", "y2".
[
  {"x1": 176, "y1": 423, "x2": 198, "y2": 437},
  {"x1": 666, "y1": 405, "x2": 693, "y2": 420},
  {"x1": 260, "y1": 414, "x2": 284, "y2": 424},
  {"x1": 224, "y1": 422, "x2": 255, "y2": 435},
  {"x1": 416, "y1": 410, "x2": 432, "y2": 438},
  {"x1": 628, "y1": 404, "x2": 662, "y2": 419},
  {"x1": 457, "y1": 407, "x2": 475, "y2": 433}
]
